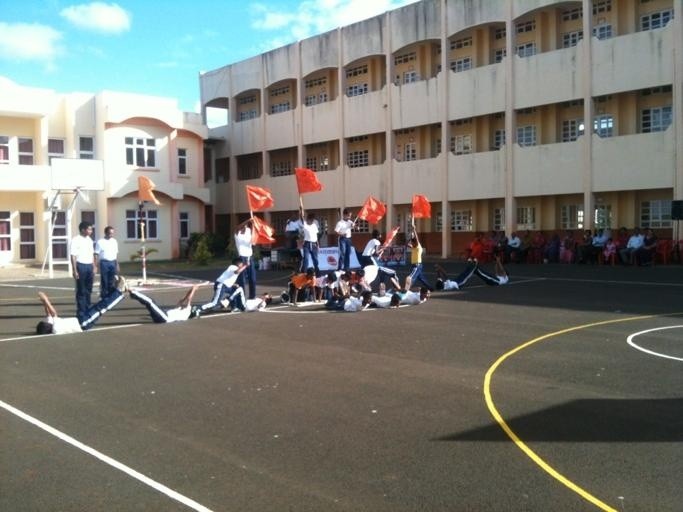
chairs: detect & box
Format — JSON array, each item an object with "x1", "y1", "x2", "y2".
[{"x1": 470, "y1": 239, "x2": 673, "y2": 264}]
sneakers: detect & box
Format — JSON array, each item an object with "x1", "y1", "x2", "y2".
[
  {"x1": 467, "y1": 257, "x2": 477, "y2": 266},
  {"x1": 195, "y1": 305, "x2": 201, "y2": 317},
  {"x1": 280, "y1": 291, "x2": 286, "y2": 302},
  {"x1": 113, "y1": 274, "x2": 129, "y2": 297},
  {"x1": 230, "y1": 307, "x2": 240, "y2": 312},
  {"x1": 219, "y1": 298, "x2": 229, "y2": 308}
]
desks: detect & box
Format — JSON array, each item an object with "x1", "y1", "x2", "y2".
[{"x1": 260, "y1": 249, "x2": 300, "y2": 272}]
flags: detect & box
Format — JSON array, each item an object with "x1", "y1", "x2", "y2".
[
  {"x1": 242, "y1": 184, "x2": 273, "y2": 213},
  {"x1": 244, "y1": 216, "x2": 274, "y2": 246},
  {"x1": 136, "y1": 176, "x2": 162, "y2": 209},
  {"x1": 293, "y1": 165, "x2": 323, "y2": 193}
]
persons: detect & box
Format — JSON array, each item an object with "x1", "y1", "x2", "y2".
[
  {"x1": 232, "y1": 213, "x2": 257, "y2": 297},
  {"x1": 34, "y1": 271, "x2": 130, "y2": 337},
  {"x1": 216, "y1": 283, "x2": 273, "y2": 314},
  {"x1": 276, "y1": 193, "x2": 433, "y2": 313},
  {"x1": 428, "y1": 223, "x2": 681, "y2": 291},
  {"x1": 92, "y1": 226, "x2": 121, "y2": 298},
  {"x1": 117, "y1": 280, "x2": 215, "y2": 325},
  {"x1": 66, "y1": 219, "x2": 98, "y2": 319},
  {"x1": 193, "y1": 254, "x2": 251, "y2": 319}
]
